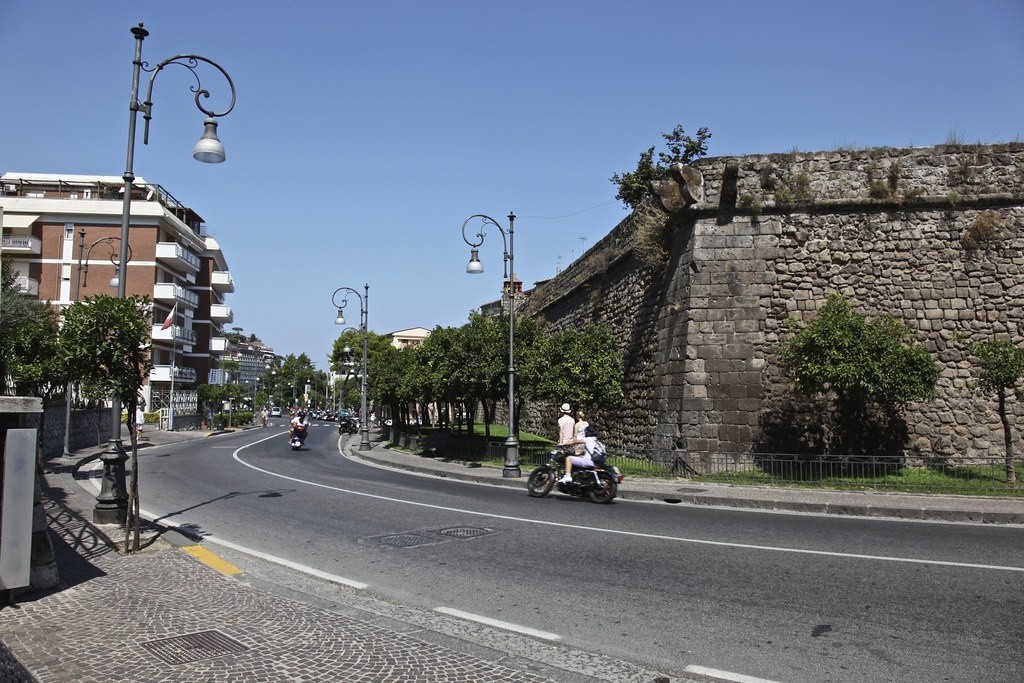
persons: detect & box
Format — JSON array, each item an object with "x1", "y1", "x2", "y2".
[
  {"x1": 136, "y1": 405, "x2": 144, "y2": 440},
  {"x1": 556, "y1": 424, "x2": 599, "y2": 484},
  {"x1": 286, "y1": 405, "x2": 309, "y2": 444},
  {"x1": 557, "y1": 402, "x2": 589, "y2": 447},
  {"x1": 261, "y1": 407, "x2": 268, "y2": 425}
]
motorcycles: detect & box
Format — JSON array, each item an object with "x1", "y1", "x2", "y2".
[
  {"x1": 338, "y1": 414, "x2": 358, "y2": 436},
  {"x1": 289, "y1": 419, "x2": 307, "y2": 451},
  {"x1": 526, "y1": 443, "x2": 624, "y2": 503}
]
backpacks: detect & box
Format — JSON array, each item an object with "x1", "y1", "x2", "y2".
[{"x1": 585, "y1": 440, "x2": 610, "y2": 466}]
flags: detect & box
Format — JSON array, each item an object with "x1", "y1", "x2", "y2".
[{"x1": 160, "y1": 304, "x2": 176, "y2": 331}]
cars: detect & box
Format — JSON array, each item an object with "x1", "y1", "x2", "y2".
[
  {"x1": 270, "y1": 406, "x2": 282, "y2": 418},
  {"x1": 308, "y1": 409, "x2": 360, "y2": 422}
]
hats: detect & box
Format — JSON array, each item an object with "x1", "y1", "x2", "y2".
[{"x1": 560, "y1": 403, "x2": 571, "y2": 413}]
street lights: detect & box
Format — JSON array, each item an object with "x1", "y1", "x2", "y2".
[
  {"x1": 462, "y1": 210, "x2": 523, "y2": 477},
  {"x1": 92, "y1": 21, "x2": 238, "y2": 525},
  {"x1": 332, "y1": 283, "x2": 372, "y2": 451},
  {"x1": 62, "y1": 226, "x2": 133, "y2": 457}
]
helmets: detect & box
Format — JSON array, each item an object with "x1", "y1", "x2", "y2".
[
  {"x1": 584, "y1": 426, "x2": 598, "y2": 437},
  {"x1": 298, "y1": 411, "x2": 305, "y2": 417}
]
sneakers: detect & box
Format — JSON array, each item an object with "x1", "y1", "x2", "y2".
[{"x1": 558, "y1": 474, "x2": 572, "y2": 484}]
